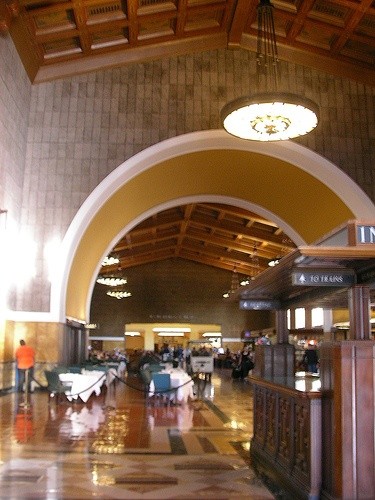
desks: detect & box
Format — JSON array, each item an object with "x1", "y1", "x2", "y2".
[
  {"x1": 58, "y1": 369, "x2": 107, "y2": 403},
  {"x1": 147, "y1": 362, "x2": 195, "y2": 405},
  {"x1": 245, "y1": 372, "x2": 325, "y2": 500},
  {"x1": 100, "y1": 362, "x2": 119, "y2": 367}
]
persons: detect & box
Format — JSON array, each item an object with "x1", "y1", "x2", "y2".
[
  {"x1": 139, "y1": 342, "x2": 232, "y2": 383},
  {"x1": 16, "y1": 340, "x2": 36, "y2": 394},
  {"x1": 87, "y1": 349, "x2": 129, "y2": 380},
  {"x1": 303, "y1": 342, "x2": 319, "y2": 374},
  {"x1": 230, "y1": 344, "x2": 257, "y2": 384}
]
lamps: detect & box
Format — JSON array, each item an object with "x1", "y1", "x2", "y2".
[
  {"x1": 220, "y1": 0, "x2": 320, "y2": 143},
  {"x1": 96, "y1": 254, "x2": 132, "y2": 300}
]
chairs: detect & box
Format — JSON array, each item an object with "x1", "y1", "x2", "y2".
[
  {"x1": 138, "y1": 358, "x2": 179, "y2": 414},
  {"x1": 44, "y1": 358, "x2": 127, "y2": 404}
]
